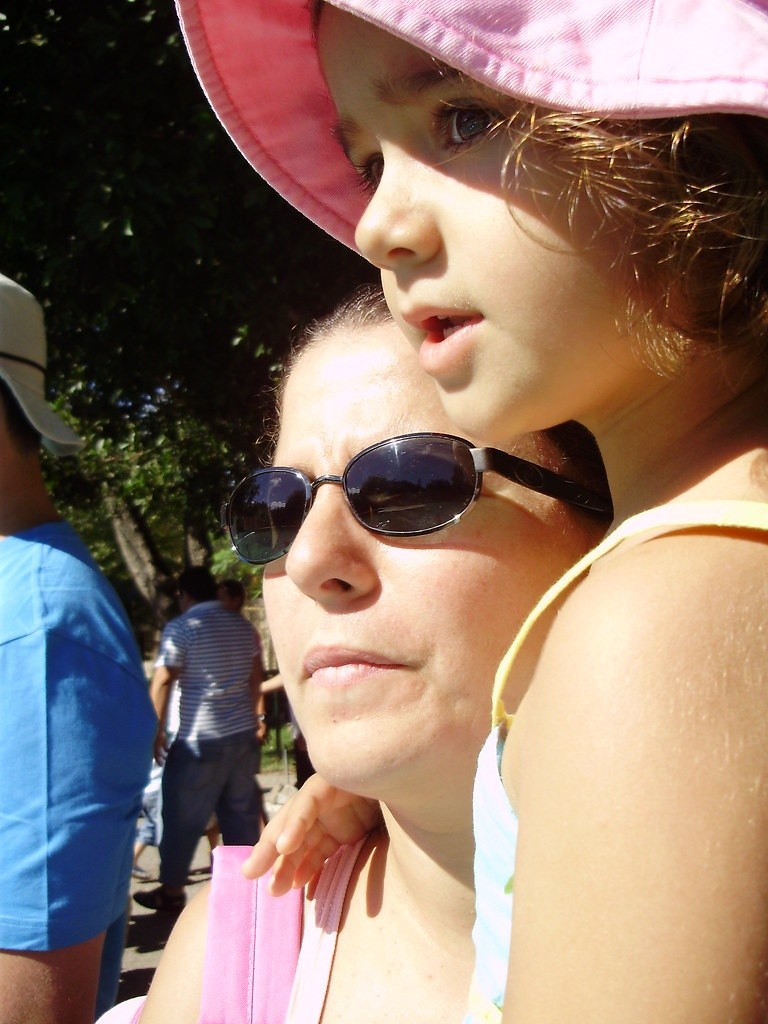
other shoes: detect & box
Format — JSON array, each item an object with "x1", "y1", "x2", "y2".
[{"x1": 131, "y1": 864, "x2": 150, "y2": 880}]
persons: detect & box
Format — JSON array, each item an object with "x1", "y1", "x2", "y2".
[
  {"x1": 131, "y1": 281, "x2": 614, "y2": 1024},
  {"x1": 0, "y1": 272, "x2": 159, "y2": 1024},
  {"x1": 176, "y1": 0, "x2": 768, "y2": 1024},
  {"x1": 196, "y1": 579, "x2": 268, "y2": 874},
  {"x1": 133, "y1": 566, "x2": 264, "y2": 913}
]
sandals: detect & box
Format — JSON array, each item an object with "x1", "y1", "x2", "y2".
[{"x1": 132, "y1": 888, "x2": 187, "y2": 913}]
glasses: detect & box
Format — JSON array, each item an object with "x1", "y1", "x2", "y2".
[{"x1": 221, "y1": 432, "x2": 614, "y2": 565}]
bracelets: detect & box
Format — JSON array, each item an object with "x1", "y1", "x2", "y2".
[{"x1": 256, "y1": 714, "x2": 268, "y2": 722}]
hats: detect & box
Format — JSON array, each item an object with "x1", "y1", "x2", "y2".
[
  {"x1": 172, "y1": 0, "x2": 768, "y2": 263},
  {"x1": 0, "y1": 275, "x2": 84, "y2": 456}
]
